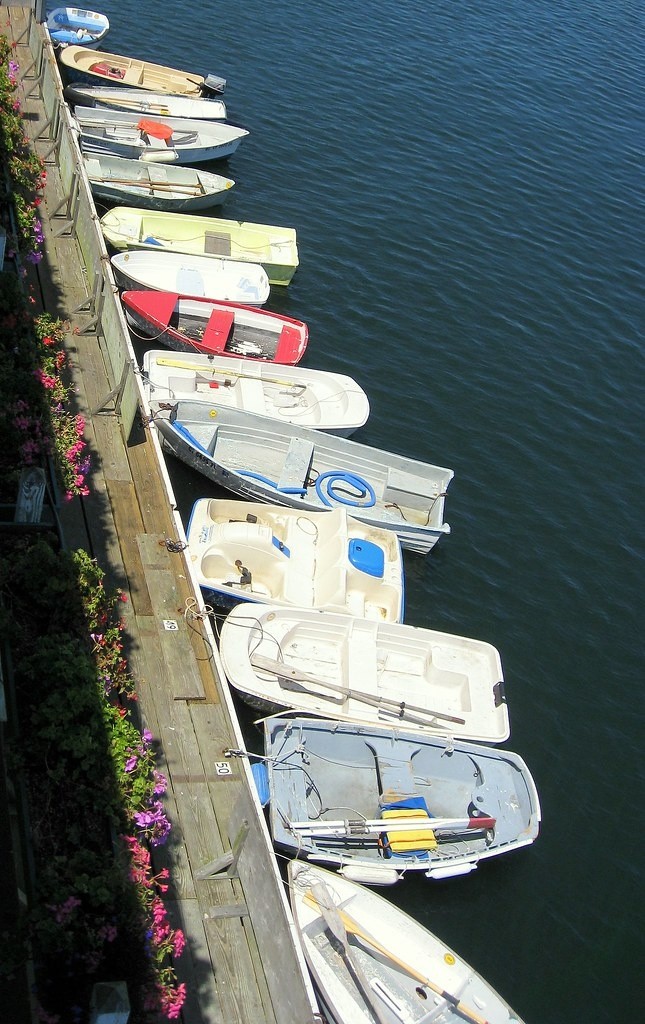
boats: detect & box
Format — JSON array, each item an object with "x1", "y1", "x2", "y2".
[
  {"x1": 119, "y1": 289, "x2": 310, "y2": 373},
  {"x1": 70, "y1": 104, "x2": 252, "y2": 166},
  {"x1": 217, "y1": 603, "x2": 512, "y2": 750},
  {"x1": 99, "y1": 206, "x2": 301, "y2": 287},
  {"x1": 251, "y1": 716, "x2": 543, "y2": 885},
  {"x1": 110, "y1": 251, "x2": 272, "y2": 315},
  {"x1": 81, "y1": 152, "x2": 237, "y2": 214},
  {"x1": 139, "y1": 347, "x2": 371, "y2": 443},
  {"x1": 185, "y1": 496, "x2": 407, "y2": 626},
  {"x1": 62, "y1": 82, "x2": 228, "y2": 125},
  {"x1": 45, "y1": 5, "x2": 111, "y2": 51},
  {"x1": 148, "y1": 397, "x2": 455, "y2": 556},
  {"x1": 286, "y1": 855, "x2": 528, "y2": 1024},
  {"x1": 59, "y1": 45, "x2": 227, "y2": 99}
]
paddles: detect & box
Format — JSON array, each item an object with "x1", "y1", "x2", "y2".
[
  {"x1": 282, "y1": 817, "x2": 497, "y2": 838},
  {"x1": 310, "y1": 880, "x2": 389, "y2": 1024},
  {"x1": 88, "y1": 176, "x2": 203, "y2": 196},
  {"x1": 94, "y1": 96, "x2": 169, "y2": 112},
  {"x1": 301, "y1": 889, "x2": 490, "y2": 1024},
  {"x1": 250, "y1": 652, "x2": 466, "y2": 731},
  {"x1": 156, "y1": 357, "x2": 307, "y2": 389}
]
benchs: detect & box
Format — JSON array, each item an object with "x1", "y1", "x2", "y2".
[
  {"x1": 84, "y1": 159, "x2": 103, "y2": 185},
  {"x1": 148, "y1": 167, "x2": 173, "y2": 198},
  {"x1": 239, "y1": 373, "x2": 267, "y2": 414},
  {"x1": 200, "y1": 308, "x2": 235, "y2": 349},
  {"x1": 146, "y1": 134, "x2": 167, "y2": 147},
  {"x1": 338, "y1": 634, "x2": 378, "y2": 721},
  {"x1": 275, "y1": 437, "x2": 315, "y2": 499},
  {"x1": 274, "y1": 326, "x2": 301, "y2": 363},
  {"x1": 123, "y1": 67, "x2": 144, "y2": 83},
  {"x1": 365, "y1": 739, "x2": 438, "y2": 862}
]
